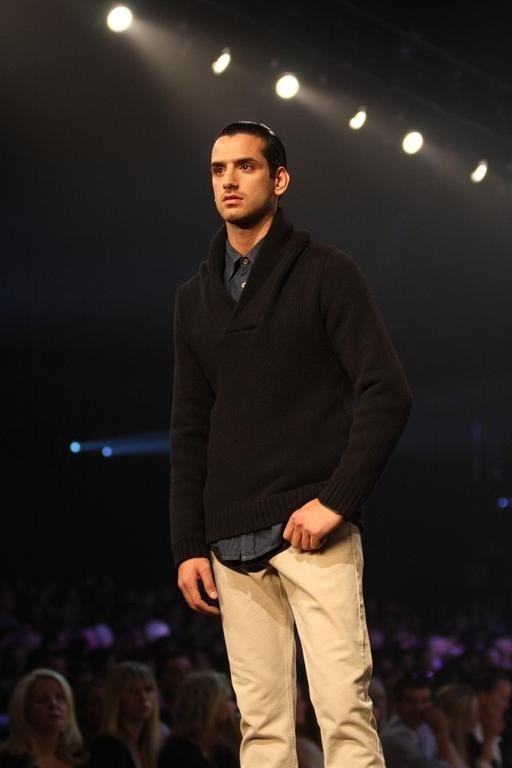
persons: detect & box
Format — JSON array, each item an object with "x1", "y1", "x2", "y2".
[
  {"x1": 166, "y1": 120, "x2": 412, "y2": 767},
  {"x1": 0, "y1": 579, "x2": 512, "y2": 767}
]
